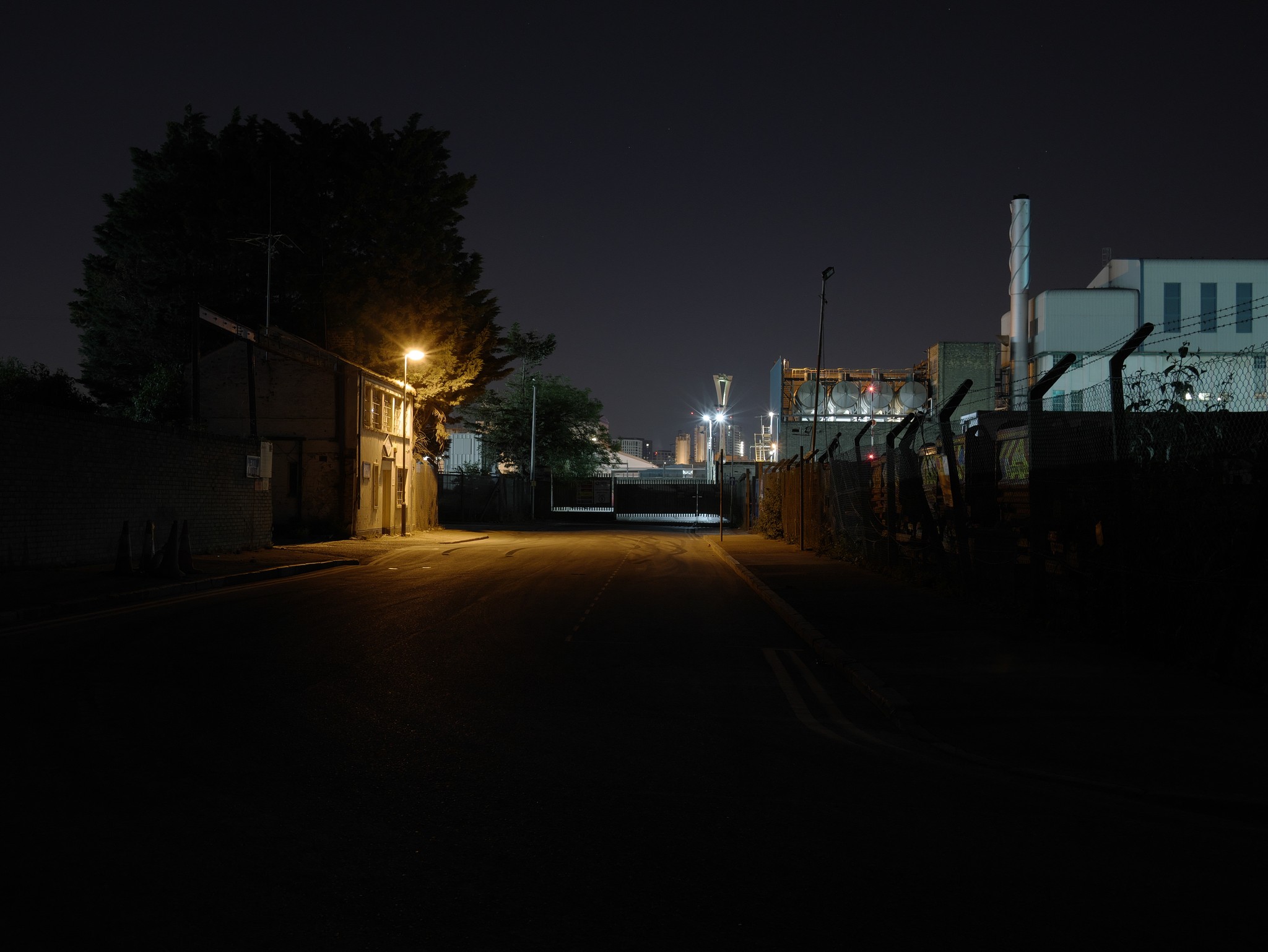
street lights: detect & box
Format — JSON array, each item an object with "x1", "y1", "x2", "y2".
[
  {"x1": 807, "y1": 266, "x2": 836, "y2": 503},
  {"x1": 401, "y1": 347, "x2": 425, "y2": 538},
  {"x1": 703, "y1": 415, "x2": 712, "y2": 484},
  {"x1": 713, "y1": 404, "x2": 734, "y2": 529},
  {"x1": 769, "y1": 412, "x2": 779, "y2": 463}
]
